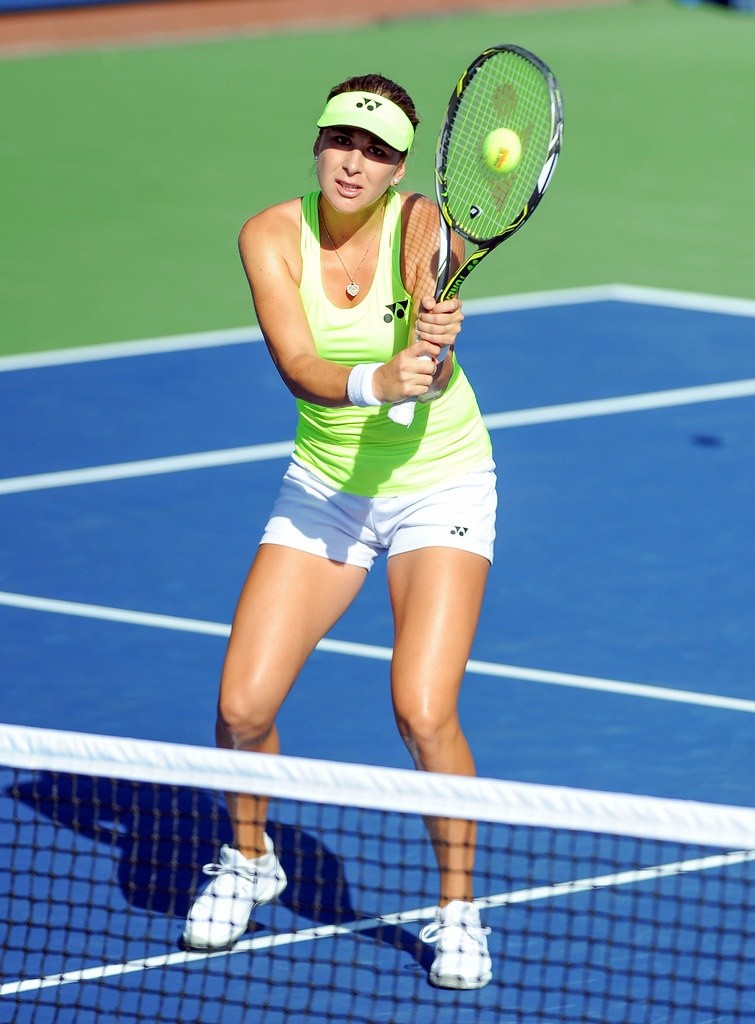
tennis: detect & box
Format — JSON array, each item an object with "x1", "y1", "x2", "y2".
[{"x1": 482, "y1": 126, "x2": 522, "y2": 175}]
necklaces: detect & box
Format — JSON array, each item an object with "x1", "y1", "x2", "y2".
[{"x1": 318, "y1": 192, "x2": 388, "y2": 297}]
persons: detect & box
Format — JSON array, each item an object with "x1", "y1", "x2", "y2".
[{"x1": 183, "y1": 73, "x2": 498, "y2": 991}]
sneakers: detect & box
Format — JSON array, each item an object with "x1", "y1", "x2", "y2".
[
  {"x1": 420, "y1": 899, "x2": 492, "y2": 990},
  {"x1": 184, "y1": 831, "x2": 287, "y2": 951}
]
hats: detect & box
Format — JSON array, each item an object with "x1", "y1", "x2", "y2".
[{"x1": 317, "y1": 90, "x2": 415, "y2": 152}]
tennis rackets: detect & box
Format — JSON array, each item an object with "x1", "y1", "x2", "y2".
[{"x1": 386, "y1": 43, "x2": 567, "y2": 428}]
